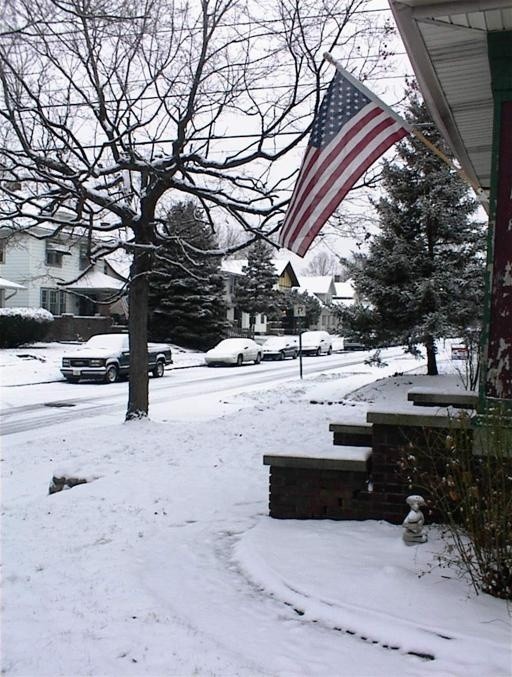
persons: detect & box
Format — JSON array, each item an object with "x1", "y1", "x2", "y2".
[{"x1": 402, "y1": 494, "x2": 429, "y2": 543}]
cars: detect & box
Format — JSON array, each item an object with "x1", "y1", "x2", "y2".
[
  {"x1": 261, "y1": 336, "x2": 300, "y2": 361},
  {"x1": 203, "y1": 337, "x2": 264, "y2": 367},
  {"x1": 300, "y1": 330, "x2": 333, "y2": 357}
]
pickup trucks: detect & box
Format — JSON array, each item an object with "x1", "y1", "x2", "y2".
[{"x1": 59, "y1": 332, "x2": 173, "y2": 385}]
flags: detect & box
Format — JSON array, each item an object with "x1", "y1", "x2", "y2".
[{"x1": 277, "y1": 68, "x2": 411, "y2": 260}]
